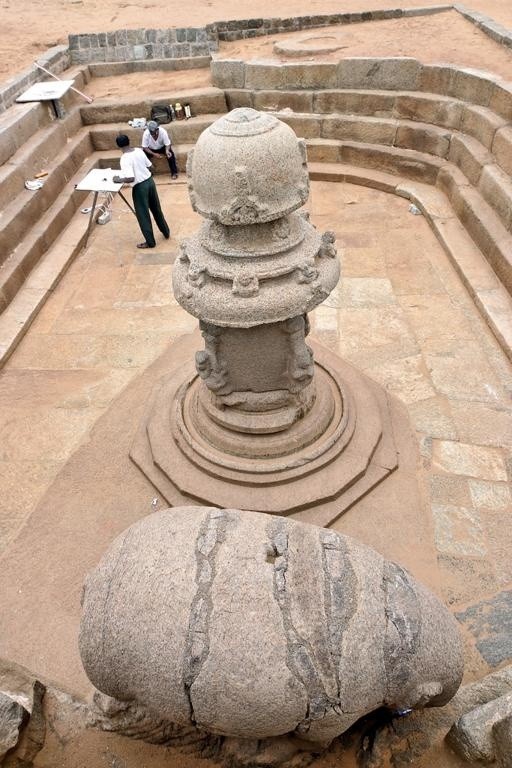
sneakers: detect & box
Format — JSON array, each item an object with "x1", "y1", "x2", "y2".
[
  {"x1": 136, "y1": 241, "x2": 148, "y2": 248},
  {"x1": 171, "y1": 173, "x2": 178, "y2": 180}
]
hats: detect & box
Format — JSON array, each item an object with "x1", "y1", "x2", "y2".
[{"x1": 115, "y1": 134, "x2": 130, "y2": 148}]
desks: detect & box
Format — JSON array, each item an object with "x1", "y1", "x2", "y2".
[{"x1": 75, "y1": 168, "x2": 139, "y2": 249}]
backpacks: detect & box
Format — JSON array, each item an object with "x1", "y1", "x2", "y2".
[{"x1": 150, "y1": 104, "x2": 173, "y2": 124}]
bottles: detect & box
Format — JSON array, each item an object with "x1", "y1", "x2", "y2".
[
  {"x1": 175, "y1": 103, "x2": 184, "y2": 120},
  {"x1": 169, "y1": 104, "x2": 176, "y2": 121},
  {"x1": 184, "y1": 103, "x2": 192, "y2": 118}
]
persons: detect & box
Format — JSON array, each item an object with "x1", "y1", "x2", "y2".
[
  {"x1": 112, "y1": 135, "x2": 170, "y2": 248},
  {"x1": 141, "y1": 120, "x2": 179, "y2": 179}
]
